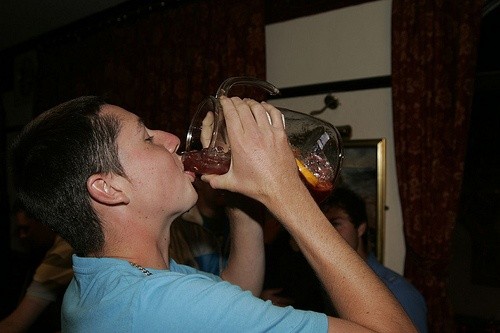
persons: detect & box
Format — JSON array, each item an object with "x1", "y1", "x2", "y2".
[
  {"x1": 4, "y1": 188, "x2": 429, "y2": 332},
  {"x1": 4, "y1": 95, "x2": 418, "y2": 332}
]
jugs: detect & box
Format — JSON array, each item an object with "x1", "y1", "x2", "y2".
[{"x1": 181, "y1": 77, "x2": 344, "y2": 198}]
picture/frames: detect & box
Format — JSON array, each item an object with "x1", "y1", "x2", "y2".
[{"x1": 338, "y1": 136, "x2": 387, "y2": 267}]
